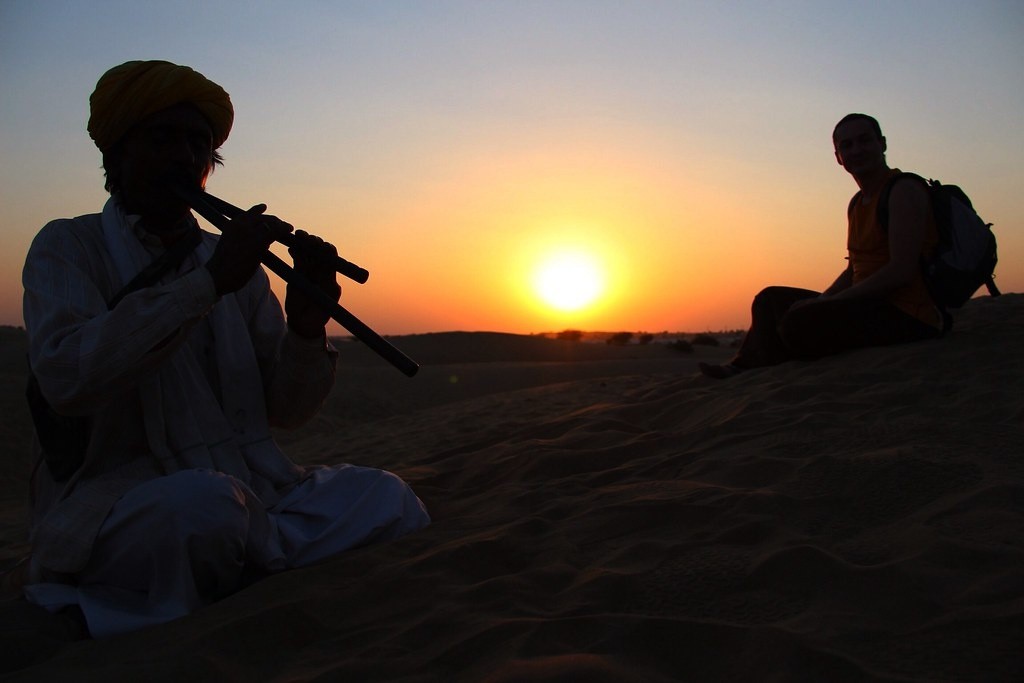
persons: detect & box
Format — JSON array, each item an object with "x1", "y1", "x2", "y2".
[
  {"x1": 697, "y1": 113, "x2": 957, "y2": 381},
  {"x1": 5, "y1": 60, "x2": 436, "y2": 642}
]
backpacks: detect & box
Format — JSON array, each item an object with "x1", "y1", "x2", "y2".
[{"x1": 848, "y1": 170, "x2": 998, "y2": 310}]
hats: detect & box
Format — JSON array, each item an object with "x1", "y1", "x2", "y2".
[{"x1": 86, "y1": 58, "x2": 235, "y2": 155}]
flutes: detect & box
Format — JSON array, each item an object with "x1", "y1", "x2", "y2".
[{"x1": 188, "y1": 185, "x2": 419, "y2": 380}]
